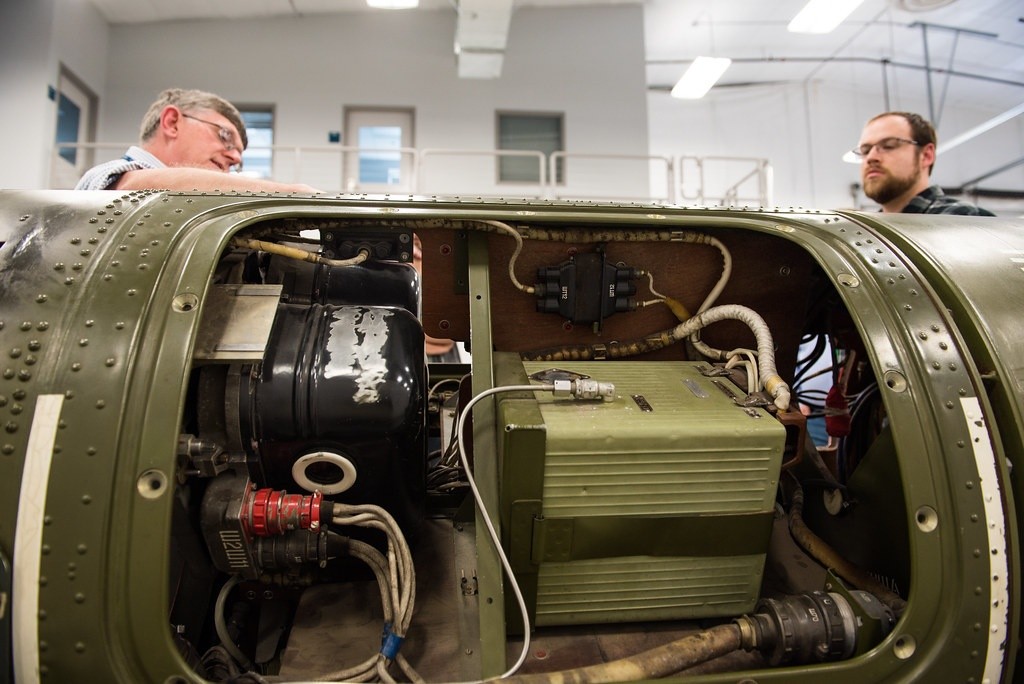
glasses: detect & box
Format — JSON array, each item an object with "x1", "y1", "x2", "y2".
[
  {"x1": 181, "y1": 114, "x2": 243, "y2": 173},
  {"x1": 852, "y1": 136, "x2": 920, "y2": 156}
]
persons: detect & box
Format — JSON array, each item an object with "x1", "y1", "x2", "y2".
[
  {"x1": 74, "y1": 88, "x2": 321, "y2": 195},
  {"x1": 853, "y1": 112, "x2": 998, "y2": 217}
]
